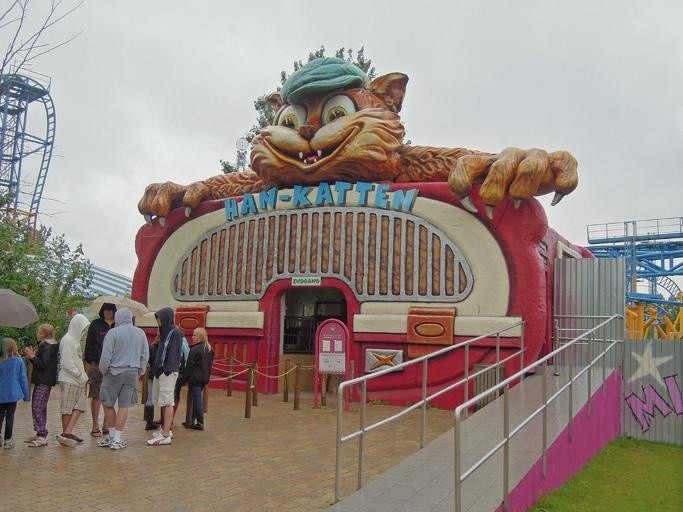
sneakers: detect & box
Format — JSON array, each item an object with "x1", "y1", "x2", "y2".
[{"x1": 4, "y1": 420, "x2": 203, "y2": 449}]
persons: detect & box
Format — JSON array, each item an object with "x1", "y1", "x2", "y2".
[
  {"x1": 96, "y1": 308, "x2": 149, "y2": 449},
  {"x1": 24, "y1": 324, "x2": 59, "y2": 447},
  {"x1": 56, "y1": 314, "x2": 92, "y2": 447},
  {"x1": 145, "y1": 308, "x2": 214, "y2": 445},
  {"x1": 85, "y1": 303, "x2": 117, "y2": 437},
  {"x1": 0, "y1": 338, "x2": 29, "y2": 449}
]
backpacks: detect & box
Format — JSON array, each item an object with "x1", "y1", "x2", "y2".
[{"x1": 161, "y1": 327, "x2": 190, "y2": 369}]
